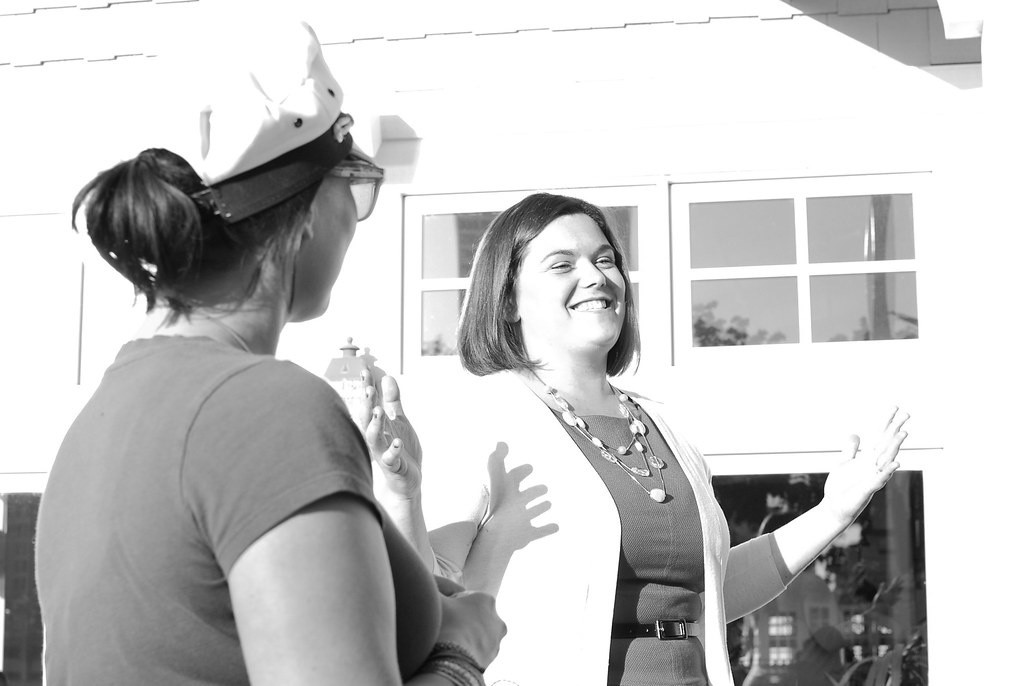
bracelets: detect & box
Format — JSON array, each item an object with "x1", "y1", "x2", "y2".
[{"x1": 420, "y1": 641, "x2": 485, "y2": 686}]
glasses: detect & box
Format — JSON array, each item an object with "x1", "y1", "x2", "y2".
[{"x1": 328, "y1": 157, "x2": 385, "y2": 218}]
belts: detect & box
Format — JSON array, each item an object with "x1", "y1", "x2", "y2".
[{"x1": 612, "y1": 620, "x2": 701, "y2": 639}]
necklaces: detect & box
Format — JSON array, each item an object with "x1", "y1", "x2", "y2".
[
  {"x1": 193, "y1": 312, "x2": 251, "y2": 354},
  {"x1": 528, "y1": 366, "x2": 666, "y2": 502}
]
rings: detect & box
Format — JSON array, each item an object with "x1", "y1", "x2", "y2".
[{"x1": 382, "y1": 461, "x2": 391, "y2": 467}]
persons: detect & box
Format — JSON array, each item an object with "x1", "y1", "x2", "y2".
[
  {"x1": 357, "y1": 193, "x2": 910, "y2": 686},
  {"x1": 32, "y1": 22, "x2": 507, "y2": 686},
  {"x1": 738, "y1": 512, "x2": 905, "y2": 686}
]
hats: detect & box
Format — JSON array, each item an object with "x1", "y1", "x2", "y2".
[{"x1": 125, "y1": 22, "x2": 344, "y2": 187}]
